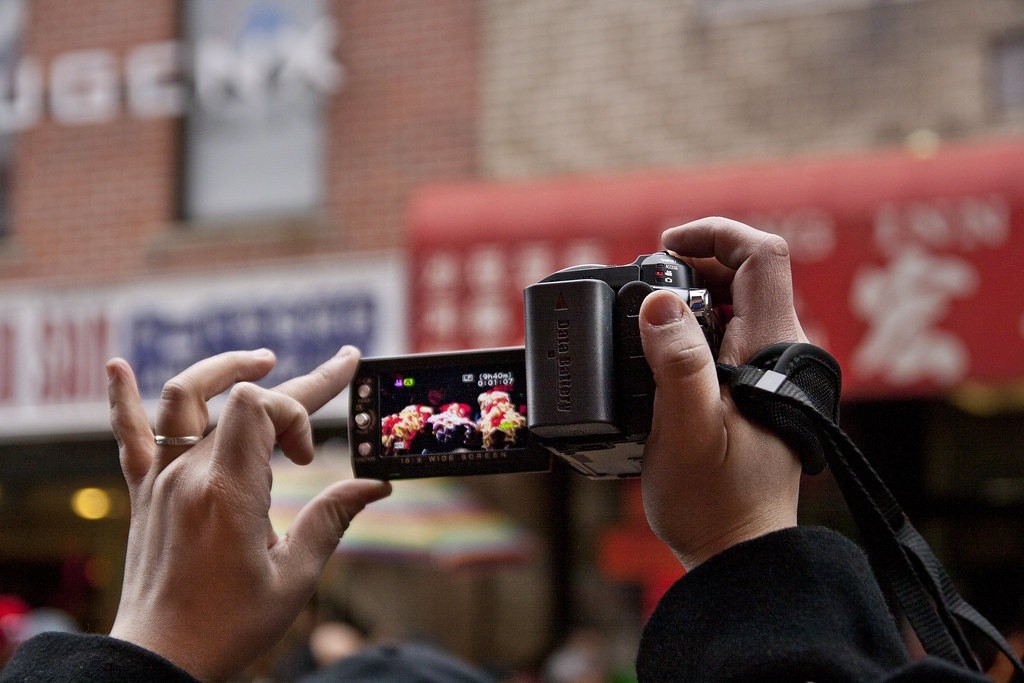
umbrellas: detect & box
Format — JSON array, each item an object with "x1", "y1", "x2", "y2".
[{"x1": 260, "y1": 450, "x2": 517, "y2": 563}]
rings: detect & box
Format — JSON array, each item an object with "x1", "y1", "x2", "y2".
[{"x1": 152, "y1": 434, "x2": 207, "y2": 447}]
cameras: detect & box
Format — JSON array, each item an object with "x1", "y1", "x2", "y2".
[{"x1": 347, "y1": 249, "x2": 727, "y2": 481}]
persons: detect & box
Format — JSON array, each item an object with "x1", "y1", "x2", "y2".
[
  {"x1": 0, "y1": 215, "x2": 998, "y2": 683},
  {"x1": 381, "y1": 390, "x2": 526, "y2": 453}
]
